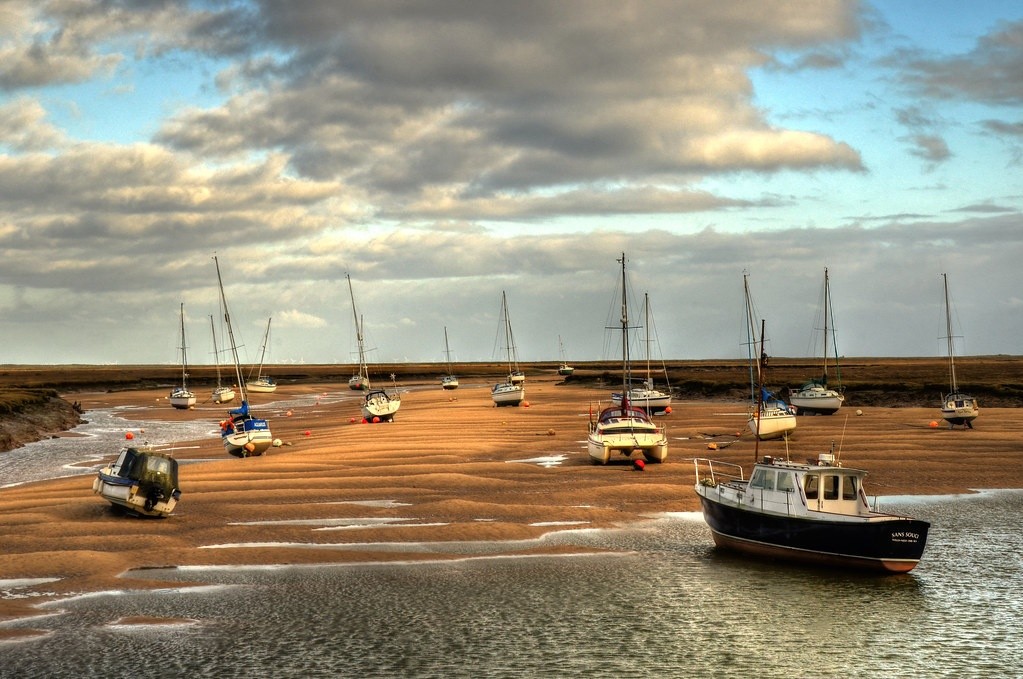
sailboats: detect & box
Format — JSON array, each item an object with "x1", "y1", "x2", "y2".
[
  {"x1": 739, "y1": 269, "x2": 797, "y2": 442},
  {"x1": 348, "y1": 313, "x2": 370, "y2": 391},
  {"x1": 209, "y1": 250, "x2": 273, "y2": 457},
  {"x1": 206, "y1": 312, "x2": 235, "y2": 404},
  {"x1": 612, "y1": 290, "x2": 672, "y2": 416},
  {"x1": 487, "y1": 291, "x2": 526, "y2": 407},
  {"x1": 786, "y1": 271, "x2": 846, "y2": 417},
  {"x1": 345, "y1": 272, "x2": 402, "y2": 422},
  {"x1": 937, "y1": 270, "x2": 980, "y2": 428},
  {"x1": 169, "y1": 302, "x2": 197, "y2": 410},
  {"x1": 244, "y1": 316, "x2": 277, "y2": 394},
  {"x1": 587, "y1": 248, "x2": 670, "y2": 466},
  {"x1": 555, "y1": 333, "x2": 574, "y2": 375},
  {"x1": 439, "y1": 326, "x2": 459, "y2": 390}
]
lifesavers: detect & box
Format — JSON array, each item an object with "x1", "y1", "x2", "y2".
[
  {"x1": 222, "y1": 421, "x2": 234, "y2": 431},
  {"x1": 973, "y1": 399, "x2": 977, "y2": 409}
]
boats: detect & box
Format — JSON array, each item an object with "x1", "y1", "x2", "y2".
[
  {"x1": 92, "y1": 438, "x2": 182, "y2": 519},
  {"x1": 694, "y1": 317, "x2": 932, "y2": 576}
]
